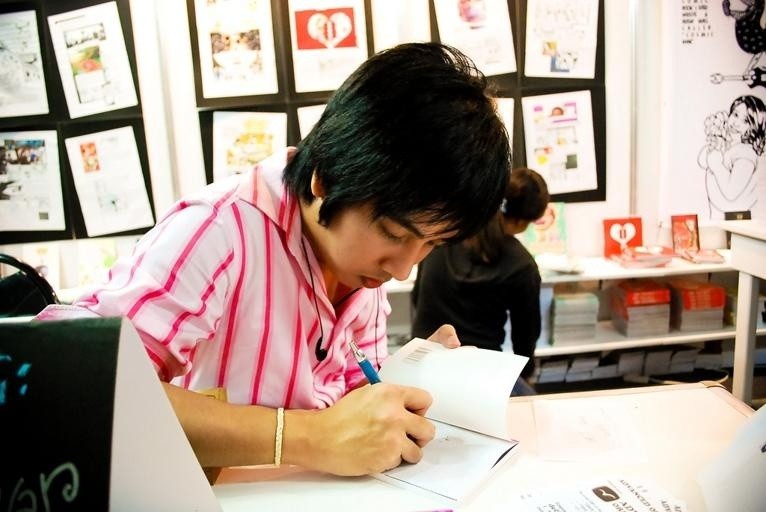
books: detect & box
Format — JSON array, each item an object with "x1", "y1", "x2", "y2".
[{"x1": 545, "y1": 277, "x2": 765, "y2": 342}]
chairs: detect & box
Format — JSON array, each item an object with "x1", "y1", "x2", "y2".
[{"x1": 0, "y1": 254, "x2": 60, "y2": 317}]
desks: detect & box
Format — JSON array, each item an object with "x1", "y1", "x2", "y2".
[
  {"x1": 717, "y1": 219, "x2": 766, "y2": 405},
  {"x1": 212, "y1": 381, "x2": 765, "y2": 512}
]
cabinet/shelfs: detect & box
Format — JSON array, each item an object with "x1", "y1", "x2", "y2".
[{"x1": 55, "y1": 248, "x2": 765, "y2": 394}]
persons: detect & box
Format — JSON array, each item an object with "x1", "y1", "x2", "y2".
[
  {"x1": 26, "y1": 42, "x2": 513, "y2": 479},
  {"x1": 697, "y1": 95, "x2": 766, "y2": 222},
  {"x1": 407, "y1": 165, "x2": 548, "y2": 396}
]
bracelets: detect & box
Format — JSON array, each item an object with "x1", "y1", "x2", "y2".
[{"x1": 270, "y1": 403, "x2": 290, "y2": 466}]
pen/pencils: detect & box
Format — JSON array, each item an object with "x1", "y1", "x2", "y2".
[{"x1": 349, "y1": 340, "x2": 381, "y2": 385}]
